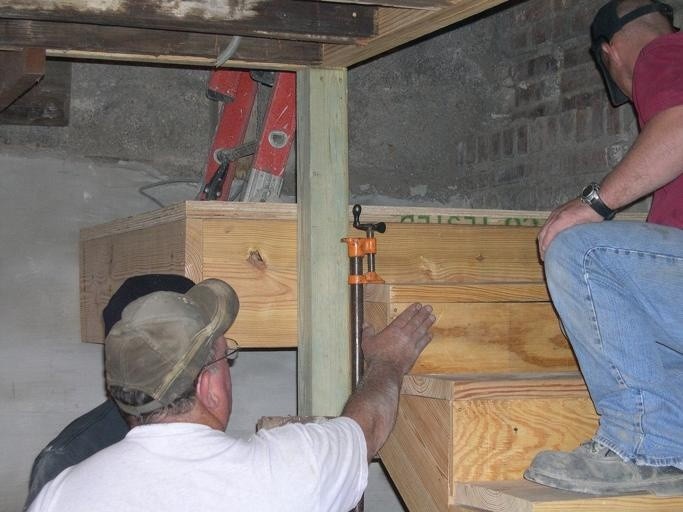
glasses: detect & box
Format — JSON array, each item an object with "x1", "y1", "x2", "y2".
[{"x1": 198, "y1": 337, "x2": 238, "y2": 372}]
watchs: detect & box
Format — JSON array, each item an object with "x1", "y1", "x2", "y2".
[{"x1": 577, "y1": 178, "x2": 614, "y2": 221}]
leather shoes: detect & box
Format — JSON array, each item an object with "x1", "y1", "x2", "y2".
[{"x1": 523, "y1": 440, "x2": 683, "y2": 498}]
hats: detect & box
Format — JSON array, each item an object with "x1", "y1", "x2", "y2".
[
  {"x1": 102, "y1": 274, "x2": 240, "y2": 417},
  {"x1": 590, "y1": 0, "x2": 674, "y2": 108}
]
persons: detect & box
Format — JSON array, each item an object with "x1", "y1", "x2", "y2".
[
  {"x1": 25, "y1": 276, "x2": 438, "y2": 512},
  {"x1": 520, "y1": 0, "x2": 682, "y2": 496},
  {"x1": 25, "y1": 275, "x2": 195, "y2": 512}
]
